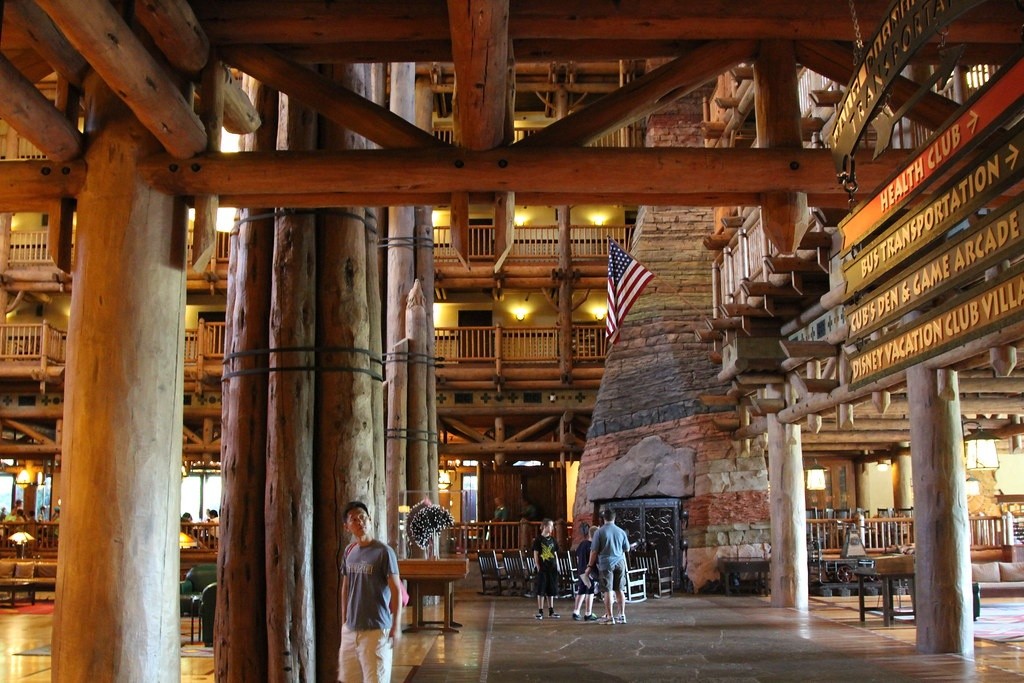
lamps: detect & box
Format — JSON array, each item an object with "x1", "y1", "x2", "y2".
[
  {"x1": 8, "y1": 531, "x2": 35, "y2": 558},
  {"x1": 965, "y1": 464, "x2": 980, "y2": 496},
  {"x1": 804, "y1": 458, "x2": 828, "y2": 491},
  {"x1": 963, "y1": 421, "x2": 1004, "y2": 470},
  {"x1": 877, "y1": 462, "x2": 890, "y2": 472}
]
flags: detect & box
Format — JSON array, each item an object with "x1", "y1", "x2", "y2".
[{"x1": 606, "y1": 238, "x2": 655, "y2": 344}]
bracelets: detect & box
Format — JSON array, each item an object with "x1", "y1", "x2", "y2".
[{"x1": 587, "y1": 565, "x2": 592, "y2": 567}]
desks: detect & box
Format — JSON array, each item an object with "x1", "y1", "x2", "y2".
[
  {"x1": 399, "y1": 558, "x2": 469, "y2": 634},
  {"x1": 854, "y1": 567, "x2": 917, "y2": 628}
]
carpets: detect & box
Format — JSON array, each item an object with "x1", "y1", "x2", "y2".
[
  {"x1": 869, "y1": 602, "x2": 1024, "y2": 642},
  {"x1": 0, "y1": 598, "x2": 55, "y2": 615},
  {"x1": 12, "y1": 617, "x2": 214, "y2": 658}
]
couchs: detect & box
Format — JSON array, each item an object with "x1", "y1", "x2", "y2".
[
  {"x1": 180, "y1": 563, "x2": 217, "y2": 647},
  {"x1": 972, "y1": 561, "x2": 1024, "y2": 597},
  {"x1": 0, "y1": 558, "x2": 57, "y2": 592}
]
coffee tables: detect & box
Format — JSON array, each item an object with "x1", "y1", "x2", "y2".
[{"x1": 0, "y1": 581, "x2": 37, "y2": 608}]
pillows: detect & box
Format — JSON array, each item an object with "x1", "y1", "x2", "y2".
[
  {"x1": 15, "y1": 560, "x2": 36, "y2": 579},
  {"x1": 0, "y1": 561, "x2": 16, "y2": 579},
  {"x1": 998, "y1": 562, "x2": 1024, "y2": 582},
  {"x1": 971, "y1": 562, "x2": 1000, "y2": 582}
]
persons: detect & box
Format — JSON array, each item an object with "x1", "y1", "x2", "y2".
[
  {"x1": 0, "y1": 499, "x2": 60, "y2": 538},
  {"x1": 572, "y1": 526, "x2": 600, "y2": 620},
  {"x1": 181, "y1": 513, "x2": 194, "y2": 534},
  {"x1": 337, "y1": 501, "x2": 402, "y2": 683},
  {"x1": 532, "y1": 519, "x2": 561, "y2": 619},
  {"x1": 521, "y1": 500, "x2": 536, "y2": 521},
  {"x1": 585, "y1": 509, "x2": 630, "y2": 625},
  {"x1": 493, "y1": 497, "x2": 508, "y2": 522},
  {"x1": 206, "y1": 510, "x2": 219, "y2": 548}
]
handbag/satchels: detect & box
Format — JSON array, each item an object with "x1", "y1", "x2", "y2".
[{"x1": 389, "y1": 579, "x2": 410, "y2": 615}]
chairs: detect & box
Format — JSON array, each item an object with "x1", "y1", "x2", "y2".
[
  {"x1": 806, "y1": 506, "x2": 915, "y2": 549},
  {"x1": 476, "y1": 549, "x2": 675, "y2": 603}
]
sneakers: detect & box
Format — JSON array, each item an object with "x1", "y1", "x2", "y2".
[
  {"x1": 614, "y1": 614, "x2": 627, "y2": 624},
  {"x1": 598, "y1": 613, "x2": 615, "y2": 625},
  {"x1": 584, "y1": 613, "x2": 599, "y2": 621},
  {"x1": 573, "y1": 612, "x2": 582, "y2": 621}
]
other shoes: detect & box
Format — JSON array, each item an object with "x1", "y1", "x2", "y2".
[
  {"x1": 536, "y1": 613, "x2": 544, "y2": 620},
  {"x1": 548, "y1": 613, "x2": 560, "y2": 618}
]
